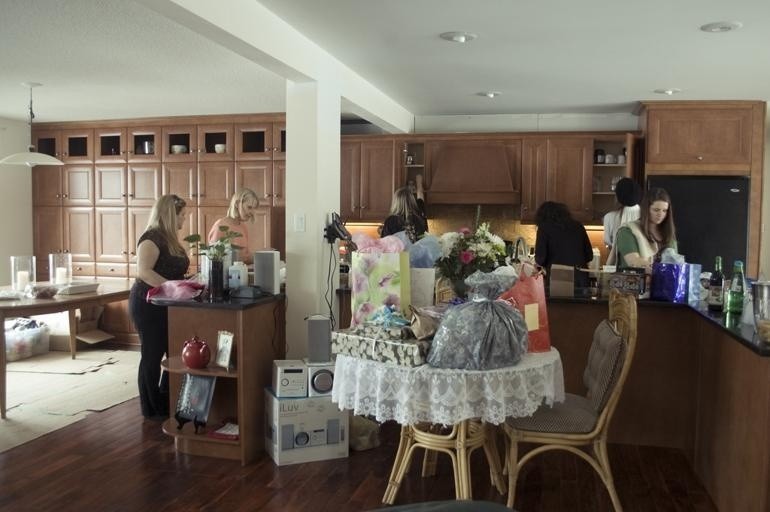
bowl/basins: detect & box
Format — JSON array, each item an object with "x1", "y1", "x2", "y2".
[
  {"x1": 170, "y1": 144, "x2": 187, "y2": 153},
  {"x1": 214, "y1": 143, "x2": 226, "y2": 154}
]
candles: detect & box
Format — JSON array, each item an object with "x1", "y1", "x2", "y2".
[
  {"x1": 17, "y1": 271, "x2": 29, "y2": 291},
  {"x1": 55, "y1": 267, "x2": 67, "y2": 287}
]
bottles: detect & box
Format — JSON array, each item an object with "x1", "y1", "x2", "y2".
[
  {"x1": 607, "y1": 175, "x2": 624, "y2": 191},
  {"x1": 143, "y1": 139, "x2": 151, "y2": 154},
  {"x1": 228, "y1": 261, "x2": 249, "y2": 289},
  {"x1": 708, "y1": 255, "x2": 725, "y2": 312},
  {"x1": 728, "y1": 261, "x2": 746, "y2": 316},
  {"x1": 407, "y1": 180, "x2": 415, "y2": 191},
  {"x1": 406, "y1": 156, "x2": 413, "y2": 165}
]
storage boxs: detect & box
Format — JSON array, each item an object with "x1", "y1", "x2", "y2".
[
  {"x1": 30, "y1": 304, "x2": 115, "y2": 352},
  {"x1": 5, "y1": 325, "x2": 49, "y2": 362},
  {"x1": 264, "y1": 387, "x2": 349, "y2": 466},
  {"x1": 330, "y1": 328, "x2": 432, "y2": 367}
]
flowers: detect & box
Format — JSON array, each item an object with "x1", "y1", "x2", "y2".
[{"x1": 417, "y1": 206, "x2": 520, "y2": 299}]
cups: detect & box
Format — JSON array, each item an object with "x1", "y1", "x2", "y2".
[
  {"x1": 10, "y1": 255, "x2": 37, "y2": 292},
  {"x1": 605, "y1": 154, "x2": 616, "y2": 164},
  {"x1": 111, "y1": 148, "x2": 117, "y2": 155},
  {"x1": 48, "y1": 253, "x2": 73, "y2": 285},
  {"x1": 618, "y1": 155, "x2": 625, "y2": 164}
]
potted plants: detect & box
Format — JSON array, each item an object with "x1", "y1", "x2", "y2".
[{"x1": 182, "y1": 226, "x2": 246, "y2": 298}]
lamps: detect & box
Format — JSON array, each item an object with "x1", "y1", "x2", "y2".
[{"x1": 0, "y1": 82, "x2": 65, "y2": 167}]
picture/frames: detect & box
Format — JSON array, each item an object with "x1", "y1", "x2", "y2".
[
  {"x1": 213, "y1": 330, "x2": 235, "y2": 372},
  {"x1": 174, "y1": 373, "x2": 217, "y2": 423}
]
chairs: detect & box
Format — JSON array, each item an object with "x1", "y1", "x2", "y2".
[
  {"x1": 502, "y1": 286, "x2": 638, "y2": 511},
  {"x1": 380, "y1": 373, "x2": 508, "y2": 505}
]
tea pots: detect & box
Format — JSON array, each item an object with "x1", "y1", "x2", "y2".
[{"x1": 181, "y1": 335, "x2": 210, "y2": 370}]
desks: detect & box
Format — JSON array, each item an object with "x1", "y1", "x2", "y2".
[
  {"x1": 331, "y1": 346, "x2": 566, "y2": 506},
  {"x1": 0, "y1": 290, "x2": 132, "y2": 419}
]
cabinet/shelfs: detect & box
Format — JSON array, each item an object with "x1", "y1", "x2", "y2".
[
  {"x1": 593, "y1": 131, "x2": 641, "y2": 225},
  {"x1": 162, "y1": 114, "x2": 235, "y2": 277},
  {"x1": 95, "y1": 116, "x2": 162, "y2": 333},
  {"x1": 521, "y1": 131, "x2": 592, "y2": 225},
  {"x1": 31, "y1": 121, "x2": 95, "y2": 282},
  {"x1": 401, "y1": 133, "x2": 429, "y2": 196},
  {"x1": 340, "y1": 133, "x2": 401, "y2": 221},
  {"x1": 234, "y1": 112, "x2": 286, "y2": 261},
  {"x1": 149, "y1": 286, "x2": 284, "y2": 467},
  {"x1": 637, "y1": 100, "x2": 752, "y2": 176}
]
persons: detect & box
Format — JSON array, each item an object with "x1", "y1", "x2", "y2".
[
  {"x1": 603, "y1": 177, "x2": 642, "y2": 266},
  {"x1": 615, "y1": 186, "x2": 678, "y2": 285},
  {"x1": 127, "y1": 193, "x2": 190, "y2": 423},
  {"x1": 533, "y1": 200, "x2": 594, "y2": 287},
  {"x1": 380, "y1": 174, "x2": 428, "y2": 244},
  {"x1": 208, "y1": 188, "x2": 259, "y2": 264}
]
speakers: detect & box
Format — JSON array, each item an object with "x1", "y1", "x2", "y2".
[
  {"x1": 254, "y1": 248, "x2": 281, "y2": 295},
  {"x1": 326, "y1": 418, "x2": 340, "y2": 445},
  {"x1": 307, "y1": 314, "x2": 330, "y2": 363},
  {"x1": 280, "y1": 423, "x2": 294, "y2": 451}
]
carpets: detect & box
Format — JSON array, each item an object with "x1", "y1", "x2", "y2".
[
  {"x1": 0, "y1": 348, "x2": 141, "y2": 455},
  {"x1": 0, "y1": 350, "x2": 167, "y2": 453}
]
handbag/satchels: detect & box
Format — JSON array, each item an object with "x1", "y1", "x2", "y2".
[
  {"x1": 688, "y1": 264, "x2": 701, "y2": 300},
  {"x1": 496, "y1": 274, "x2": 550, "y2": 353},
  {"x1": 410, "y1": 268, "x2": 435, "y2": 306},
  {"x1": 650, "y1": 262, "x2": 688, "y2": 303},
  {"x1": 350, "y1": 251, "x2": 410, "y2": 331}
]
